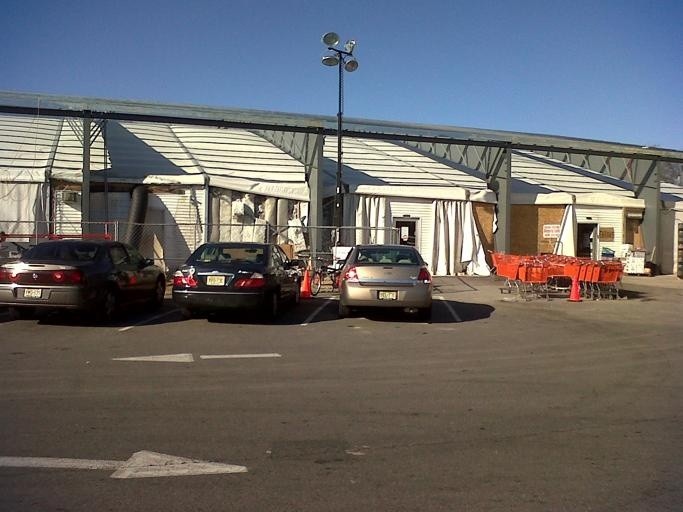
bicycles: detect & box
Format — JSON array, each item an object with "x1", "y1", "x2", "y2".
[{"x1": 309, "y1": 256, "x2": 346, "y2": 296}]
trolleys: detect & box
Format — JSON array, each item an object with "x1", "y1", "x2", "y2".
[{"x1": 490, "y1": 251, "x2": 629, "y2": 302}]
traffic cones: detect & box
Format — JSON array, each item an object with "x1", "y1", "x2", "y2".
[{"x1": 300, "y1": 268, "x2": 311, "y2": 298}]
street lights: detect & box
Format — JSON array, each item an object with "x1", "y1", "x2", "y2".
[{"x1": 322, "y1": 30, "x2": 360, "y2": 247}]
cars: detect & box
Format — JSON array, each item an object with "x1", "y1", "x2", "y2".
[
  {"x1": 0, "y1": 240, "x2": 167, "y2": 323},
  {"x1": 0, "y1": 241, "x2": 28, "y2": 266},
  {"x1": 337, "y1": 243, "x2": 433, "y2": 318},
  {"x1": 172, "y1": 242, "x2": 302, "y2": 319}
]
